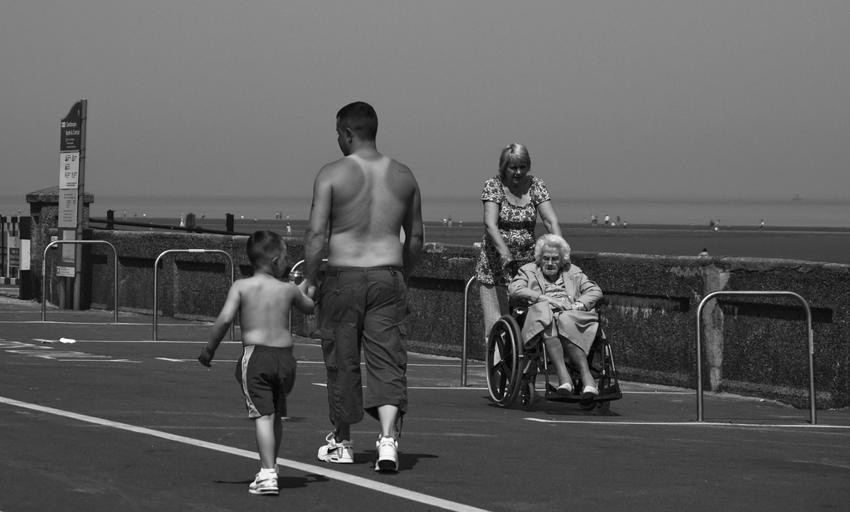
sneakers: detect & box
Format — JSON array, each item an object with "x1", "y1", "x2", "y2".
[
  {"x1": 317, "y1": 432, "x2": 354, "y2": 464},
  {"x1": 248, "y1": 472, "x2": 280, "y2": 495},
  {"x1": 580, "y1": 386, "x2": 600, "y2": 400},
  {"x1": 557, "y1": 383, "x2": 575, "y2": 393},
  {"x1": 374, "y1": 440, "x2": 399, "y2": 473}
]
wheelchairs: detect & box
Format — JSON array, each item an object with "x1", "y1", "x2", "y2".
[{"x1": 484, "y1": 260, "x2": 624, "y2": 417}]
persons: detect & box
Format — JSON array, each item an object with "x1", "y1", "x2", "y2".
[{"x1": 476, "y1": 143, "x2": 605, "y2": 396}]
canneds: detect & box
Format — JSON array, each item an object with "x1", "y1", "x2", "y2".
[{"x1": 289, "y1": 270, "x2": 302, "y2": 285}]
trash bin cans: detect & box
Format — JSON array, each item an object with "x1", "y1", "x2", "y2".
[{"x1": 288, "y1": 271, "x2": 318, "y2": 337}]
